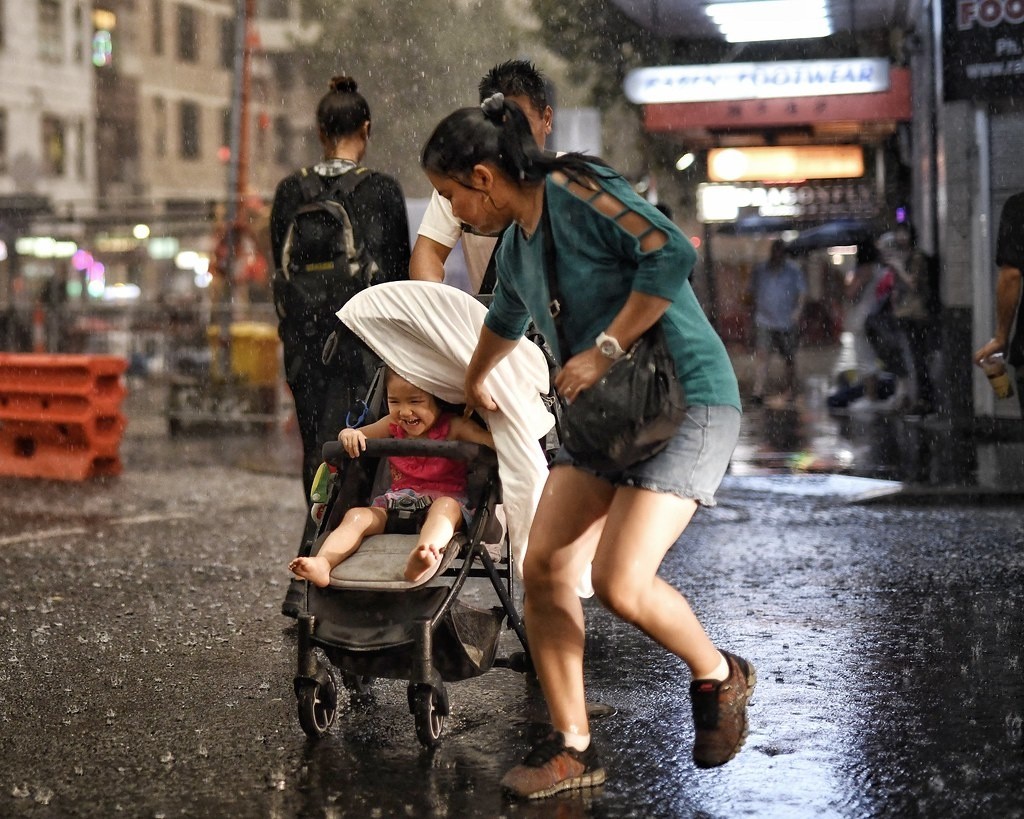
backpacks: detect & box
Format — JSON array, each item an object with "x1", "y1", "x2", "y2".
[{"x1": 272, "y1": 163, "x2": 380, "y2": 387}]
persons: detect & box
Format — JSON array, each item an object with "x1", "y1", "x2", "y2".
[
  {"x1": 268, "y1": 77, "x2": 412, "y2": 616},
  {"x1": 748, "y1": 242, "x2": 805, "y2": 404},
  {"x1": 408, "y1": 57, "x2": 568, "y2": 298},
  {"x1": 824, "y1": 223, "x2": 941, "y2": 417},
  {"x1": 286, "y1": 361, "x2": 496, "y2": 587},
  {"x1": 419, "y1": 95, "x2": 756, "y2": 797},
  {"x1": 973, "y1": 191, "x2": 1024, "y2": 420}
]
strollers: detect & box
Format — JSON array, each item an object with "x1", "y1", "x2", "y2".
[{"x1": 280, "y1": 277, "x2": 562, "y2": 747}]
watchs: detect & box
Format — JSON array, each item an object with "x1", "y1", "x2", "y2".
[{"x1": 594, "y1": 331, "x2": 626, "y2": 362}]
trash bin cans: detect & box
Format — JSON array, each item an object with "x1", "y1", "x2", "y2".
[{"x1": 204, "y1": 321, "x2": 281, "y2": 393}]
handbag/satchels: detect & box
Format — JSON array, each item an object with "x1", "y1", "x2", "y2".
[{"x1": 564, "y1": 336, "x2": 690, "y2": 480}]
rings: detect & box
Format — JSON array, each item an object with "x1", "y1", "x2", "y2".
[{"x1": 567, "y1": 385, "x2": 573, "y2": 392}]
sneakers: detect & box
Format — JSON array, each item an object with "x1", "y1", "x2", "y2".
[
  {"x1": 280, "y1": 578, "x2": 307, "y2": 617},
  {"x1": 498, "y1": 732, "x2": 607, "y2": 800},
  {"x1": 687, "y1": 648, "x2": 758, "y2": 769}
]
